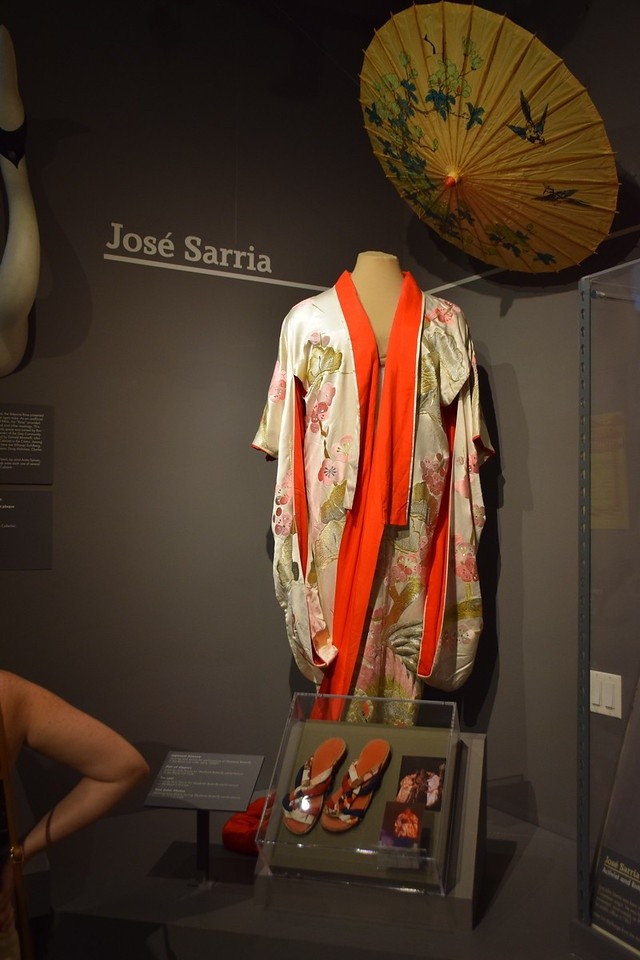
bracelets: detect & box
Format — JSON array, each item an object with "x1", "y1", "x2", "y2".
[{"x1": 9, "y1": 844, "x2": 24, "y2": 863}]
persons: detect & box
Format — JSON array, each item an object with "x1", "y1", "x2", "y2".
[
  {"x1": 249, "y1": 251, "x2": 497, "y2": 720},
  {"x1": 0, "y1": 670, "x2": 151, "y2": 960},
  {"x1": 393, "y1": 767, "x2": 441, "y2": 838}
]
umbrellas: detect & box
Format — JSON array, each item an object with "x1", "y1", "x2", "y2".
[{"x1": 358, "y1": 0, "x2": 621, "y2": 275}]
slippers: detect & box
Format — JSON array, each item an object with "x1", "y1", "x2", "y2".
[
  {"x1": 281, "y1": 736, "x2": 347, "y2": 835},
  {"x1": 321, "y1": 737, "x2": 390, "y2": 832}
]
